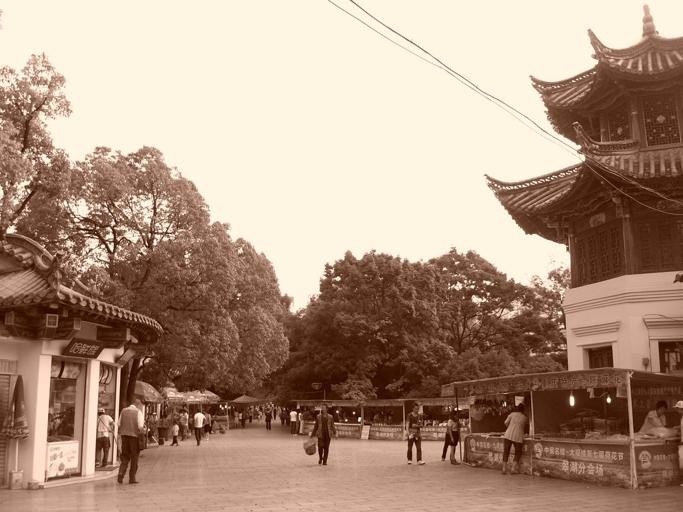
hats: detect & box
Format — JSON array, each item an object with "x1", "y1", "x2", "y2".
[{"x1": 99, "y1": 408, "x2": 106, "y2": 413}]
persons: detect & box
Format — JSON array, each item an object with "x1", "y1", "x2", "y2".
[
  {"x1": 231, "y1": 402, "x2": 467, "y2": 436},
  {"x1": 146, "y1": 403, "x2": 214, "y2": 447},
  {"x1": 500, "y1": 403, "x2": 528, "y2": 476},
  {"x1": 637, "y1": 401, "x2": 668, "y2": 436},
  {"x1": 441, "y1": 409, "x2": 461, "y2": 465},
  {"x1": 95, "y1": 407, "x2": 115, "y2": 468},
  {"x1": 403, "y1": 403, "x2": 425, "y2": 466},
  {"x1": 671, "y1": 400, "x2": 683, "y2": 487},
  {"x1": 309, "y1": 403, "x2": 337, "y2": 466},
  {"x1": 116, "y1": 399, "x2": 147, "y2": 484}
]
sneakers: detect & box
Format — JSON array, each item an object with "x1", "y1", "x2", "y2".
[
  {"x1": 417, "y1": 460, "x2": 426, "y2": 465},
  {"x1": 95, "y1": 463, "x2": 100, "y2": 467},
  {"x1": 408, "y1": 460, "x2": 412, "y2": 464},
  {"x1": 129, "y1": 479, "x2": 139, "y2": 483},
  {"x1": 319, "y1": 458, "x2": 328, "y2": 465},
  {"x1": 442, "y1": 457, "x2": 461, "y2": 465},
  {"x1": 118, "y1": 475, "x2": 122, "y2": 483}
]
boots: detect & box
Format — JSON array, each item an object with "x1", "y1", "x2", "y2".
[{"x1": 502, "y1": 459, "x2": 520, "y2": 475}]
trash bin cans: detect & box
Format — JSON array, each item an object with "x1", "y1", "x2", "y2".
[{"x1": 158, "y1": 427, "x2": 168, "y2": 441}]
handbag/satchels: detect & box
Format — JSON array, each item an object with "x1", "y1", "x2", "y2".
[
  {"x1": 138, "y1": 433, "x2": 147, "y2": 450},
  {"x1": 304, "y1": 440, "x2": 316, "y2": 455}
]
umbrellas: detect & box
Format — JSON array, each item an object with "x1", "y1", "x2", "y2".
[{"x1": 0, "y1": 374, "x2": 31, "y2": 472}]
